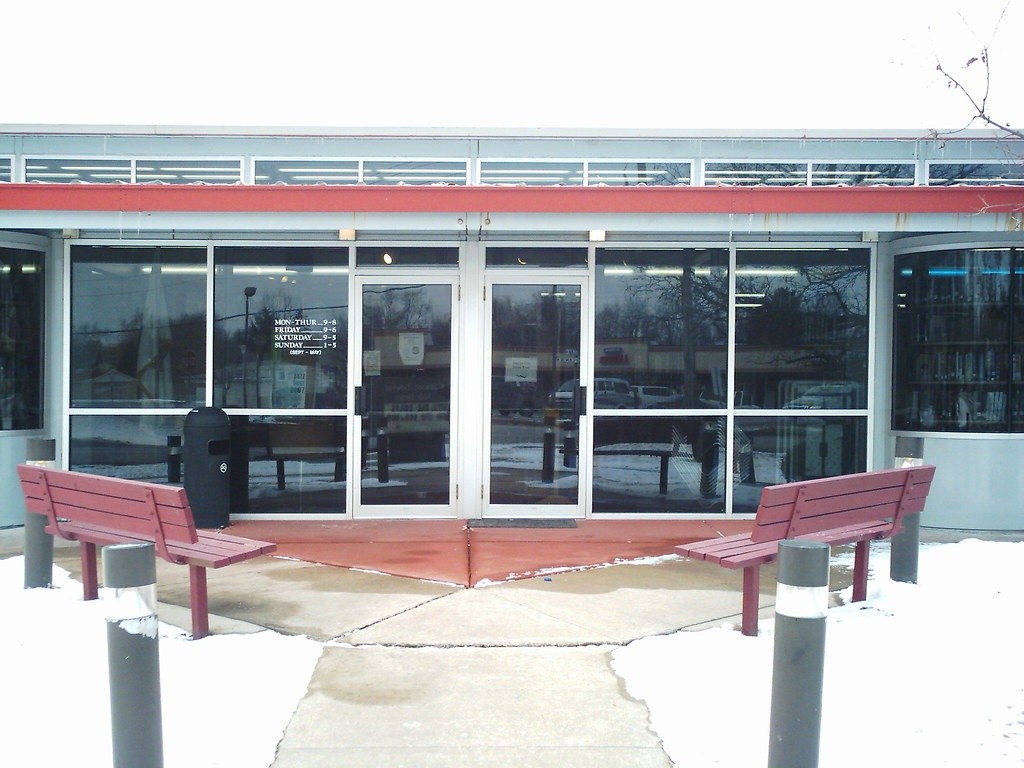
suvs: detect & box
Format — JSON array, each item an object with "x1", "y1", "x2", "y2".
[{"x1": 630, "y1": 385, "x2": 687, "y2": 409}]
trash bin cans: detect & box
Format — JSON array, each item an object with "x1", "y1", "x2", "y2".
[{"x1": 182, "y1": 407, "x2": 233, "y2": 528}]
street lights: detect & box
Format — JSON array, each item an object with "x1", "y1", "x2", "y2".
[{"x1": 242, "y1": 287, "x2": 258, "y2": 407}]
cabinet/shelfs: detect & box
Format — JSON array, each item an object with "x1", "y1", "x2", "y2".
[{"x1": 913, "y1": 266, "x2": 1024, "y2": 433}]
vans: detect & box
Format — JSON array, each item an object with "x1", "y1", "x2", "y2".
[{"x1": 548, "y1": 377, "x2": 635, "y2": 420}]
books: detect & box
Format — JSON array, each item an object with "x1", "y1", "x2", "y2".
[{"x1": 911, "y1": 265, "x2": 1024, "y2": 423}]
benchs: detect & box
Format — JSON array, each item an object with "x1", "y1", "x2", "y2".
[
  {"x1": 674, "y1": 465, "x2": 937, "y2": 637},
  {"x1": 17, "y1": 463, "x2": 276, "y2": 640},
  {"x1": 594, "y1": 415, "x2": 699, "y2": 494},
  {"x1": 248, "y1": 422, "x2": 346, "y2": 490}
]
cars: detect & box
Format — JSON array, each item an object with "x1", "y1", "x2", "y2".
[{"x1": 781, "y1": 384, "x2": 867, "y2": 411}]
